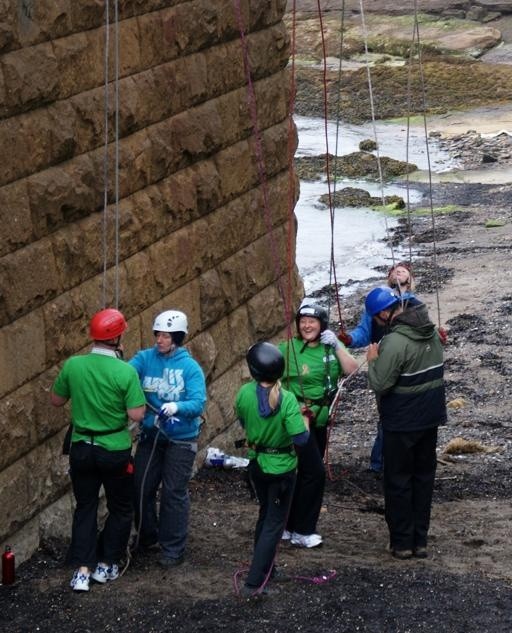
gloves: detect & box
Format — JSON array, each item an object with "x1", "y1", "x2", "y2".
[
  {"x1": 337, "y1": 333, "x2": 352, "y2": 346},
  {"x1": 319, "y1": 330, "x2": 338, "y2": 347},
  {"x1": 158, "y1": 401, "x2": 178, "y2": 419},
  {"x1": 437, "y1": 327, "x2": 447, "y2": 344}
]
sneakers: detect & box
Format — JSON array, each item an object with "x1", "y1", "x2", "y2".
[
  {"x1": 91, "y1": 564, "x2": 120, "y2": 583},
  {"x1": 281, "y1": 530, "x2": 323, "y2": 540},
  {"x1": 290, "y1": 532, "x2": 323, "y2": 549},
  {"x1": 158, "y1": 555, "x2": 183, "y2": 567},
  {"x1": 387, "y1": 543, "x2": 414, "y2": 559},
  {"x1": 69, "y1": 571, "x2": 92, "y2": 592},
  {"x1": 414, "y1": 546, "x2": 428, "y2": 557}
]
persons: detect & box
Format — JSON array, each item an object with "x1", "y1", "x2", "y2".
[
  {"x1": 48, "y1": 308, "x2": 150, "y2": 593},
  {"x1": 276, "y1": 303, "x2": 360, "y2": 549},
  {"x1": 366, "y1": 285, "x2": 446, "y2": 561},
  {"x1": 116, "y1": 311, "x2": 207, "y2": 568},
  {"x1": 337, "y1": 265, "x2": 448, "y2": 478},
  {"x1": 233, "y1": 343, "x2": 311, "y2": 589}
]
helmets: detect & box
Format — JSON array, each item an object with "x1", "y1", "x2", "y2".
[
  {"x1": 89, "y1": 308, "x2": 128, "y2": 341},
  {"x1": 247, "y1": 342, "x2": 285, "y2": 382},
  {"x1": 364, "y1": 287, "x2": 398, "y2": 316},
  {"x1": 295, "y1": 304, "x2": 329, "y2": 333},
  {"x1": 153, "y1": 310, "x2": 189, "y2": 336}
]
